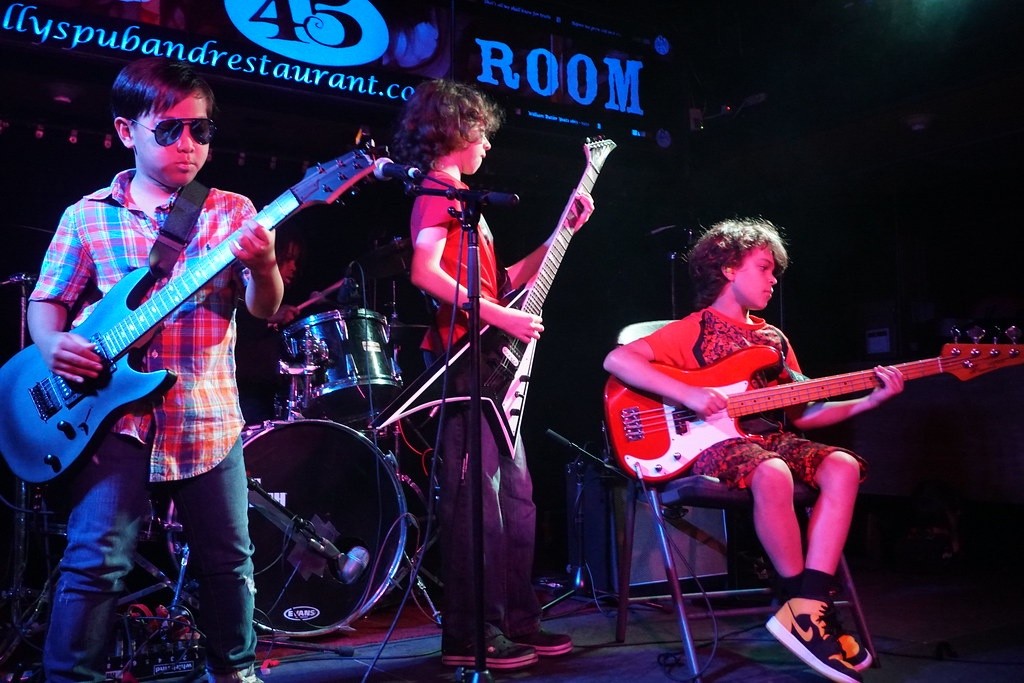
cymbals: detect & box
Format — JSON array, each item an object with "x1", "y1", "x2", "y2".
[
  {"x1": 385, "y1": 318, "x2": 430, "y2": 329},
  {"x1": 347, "y1": 237, "x2": 413, "y2": 280}
]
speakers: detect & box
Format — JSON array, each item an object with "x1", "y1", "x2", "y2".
[{"x1": 565, "y1": 463, "x2": 732, "y2": 600}]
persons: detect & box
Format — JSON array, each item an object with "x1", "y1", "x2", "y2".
[
  {"x1": 234, "y1": 227, "x2": 299, "y2": 423},
  {"x1": 392, "y1": 79, "x2": 596, "y2": 668},
  {"x1": 27, "y1": 56, "x2": 285, "y2": 683},
  {"x1": 604, "y1": 218, "x2": 903, "y2": 683}
]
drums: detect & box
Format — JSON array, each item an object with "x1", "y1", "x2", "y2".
[
  {"x1": 273, "y1": 306, "x2": 404, "y2": 423},
  {"x1": 165, "y1": 419, "x2": 410, "y2": 635}
]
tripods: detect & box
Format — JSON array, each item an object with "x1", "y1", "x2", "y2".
[{"x1": 540, "y1": 429, "x2": 644, "y2": 610}]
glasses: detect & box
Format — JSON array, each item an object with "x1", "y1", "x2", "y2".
[{"x1": 130, "y1": 117, "x2": 216, "y2": 148}]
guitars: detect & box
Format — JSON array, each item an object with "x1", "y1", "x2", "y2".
[
  {"x1": 603, "y1": 326, "x2": 1024, "y2": 483},
  {"x1": 0, "y1": 146, "x2": 383, "y2": 501},
  {"x1": 366, "y1": 135, "x2": 617, "y2": 463}
]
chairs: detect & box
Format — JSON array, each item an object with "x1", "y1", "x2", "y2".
[{"x1": 615, "y1": 320, "x2": 880, "y2": 683}]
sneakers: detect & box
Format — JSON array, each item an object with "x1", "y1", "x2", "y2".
[
  {"x1": 444, "y1": 636, "x2": 538, "y2": 670},
  {"x1": 766, "y1": 598, "x2": 872, "y2": 683},
  {"x1": 506, "y1": 631, "x2": 573, "y2": 656}
]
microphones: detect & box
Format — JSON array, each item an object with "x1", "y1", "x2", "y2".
[
  {"x1": 373, "y1": 157, "x2": 420, "y2": 182},
  {"x1": 331, "y1": 546, "x2": 371, "y2": 585},
  {"x1": 660, "y1": 508, "x2": 690, "y2": 519},
  {"x1": 337, "y1": 264, "x2": 353, "y2": 304}
]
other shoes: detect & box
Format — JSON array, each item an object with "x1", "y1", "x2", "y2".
[{"x1": 218, "y1": 665, "x2": 264, "y2": 683}]
